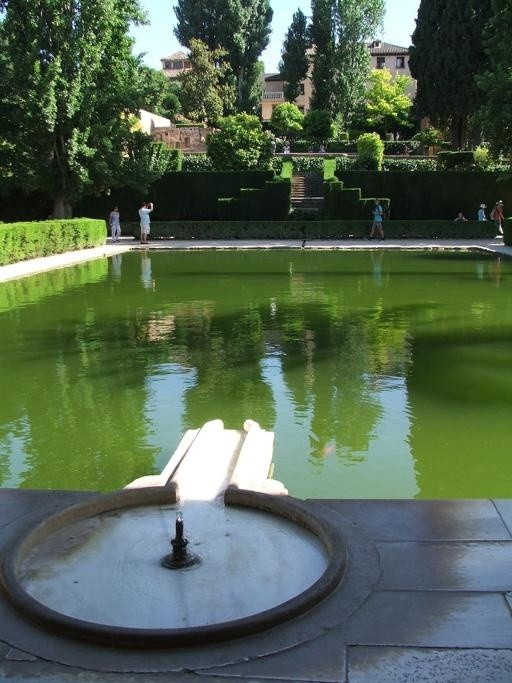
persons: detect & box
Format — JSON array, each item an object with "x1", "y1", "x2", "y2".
[
  {"x1": 108, "y1": 205, "x2": 121, "y2": 242},
  {"x1": 141, "y1": 248, "x2": 153, "y2": 293},
  {"x1": 489, "y1": 201, "x2": 498, "y2": 220},
  {"x1": 493, "y1": 199, "x2": 505, "y2": 234},
  {"x1": 475, "y1": 252, "x2": 484, "y2": 280},
  {"x1": 476, "y1": 203, "x2": 488, "y2": 221},
  {"x1": 454, "y1": 210, "x2": 468, "y2": 222},
  {"x1": 138, "y1": 201, "x2": 154, "y2": 244},
  {"x1": 369, "y1": 249, "x2": 384, "y2": 303},
  {"x1": 366, "y1": 197, "x2": 386, "y2": 242},
  {"x1": 488, "y1": 255, "x2": 503, "y2": 288},
  {"x1": 110, "y1": 254, "x2": 123, "y2": 282}
]
